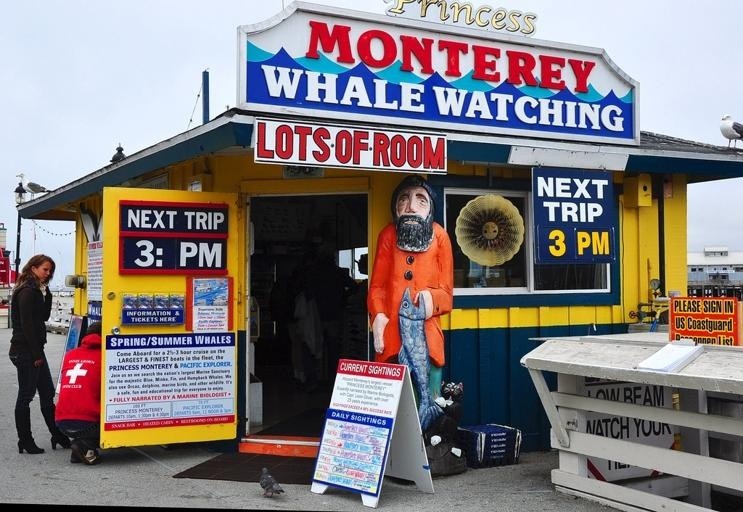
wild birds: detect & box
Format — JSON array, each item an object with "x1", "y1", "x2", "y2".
[
  {"x1": 16, "y1": 172, "x2": 52, "y2": 201},
  {"x1": 719, "y1": 113, "x2": 743, "y2": 148}
]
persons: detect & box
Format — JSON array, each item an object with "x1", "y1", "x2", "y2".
[
  {"x1": 368, "y1": 175, "x2": 454, "y2": 412},
  {"x1": 8, "y1": 254, "x2": 101, "y2": 465}
]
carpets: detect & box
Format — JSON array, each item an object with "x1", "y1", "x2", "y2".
[{"x1": 173, "y1": 452, "x2": 318, "y2": 485}]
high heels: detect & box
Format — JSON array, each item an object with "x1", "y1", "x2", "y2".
[
  {"x1": 18, "y1": 438, "x2": 44, "y2": 454},
  {"x1": 51, "y1": 432, "x2": 72, "y2": 449}
]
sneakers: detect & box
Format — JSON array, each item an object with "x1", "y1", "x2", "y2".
[{"x1": 70, "y1": 438, "x2": 99, "y2": 464}]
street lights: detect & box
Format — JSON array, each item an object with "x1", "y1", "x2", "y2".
[{"x1": 9, "y1": 180, "x2": 26, "y2": 283}]
